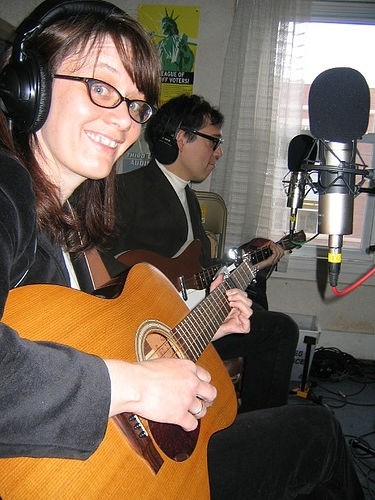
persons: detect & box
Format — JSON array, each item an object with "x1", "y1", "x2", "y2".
[
  {"x1": 1, "y1": 0, "x2": 364, "y2": 500},
  {"x1": 95, "y1": 94, "x2": 300, "y2": 413}
]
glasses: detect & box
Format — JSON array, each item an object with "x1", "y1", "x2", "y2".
[
  {"x1": 182, "y1": 127, "x2": 225, "y2": 152},
  {"x1": 52, "y1": 73, "x2": 156, "y2": 125}
]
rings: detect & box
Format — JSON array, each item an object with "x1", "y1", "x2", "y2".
[{"x1": 193, "y1": 400, "x2": 204, "y2": 415}]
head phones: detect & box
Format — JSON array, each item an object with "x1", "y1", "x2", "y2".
[
  {"x1": 154, "y1": 96, "x2": 204, "y2": 165},
  {"x1": 0, "y1": 0, "x2": 129, "y2": 135}
]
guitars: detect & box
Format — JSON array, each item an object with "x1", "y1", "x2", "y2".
[
  {"x1": 0, "y1": 248, "x2": 259, "y2": 500},
  {"x1": 113, "y1": 230, "x2": 307, "y2": 309}
]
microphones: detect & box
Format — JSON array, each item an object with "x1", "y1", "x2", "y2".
[
  {"x1": 288, "y1": 134, "x2": 317, "y2": 233},
  {"x1": 308, "y1": 67, "x2": 371, "y2": 287}
]
